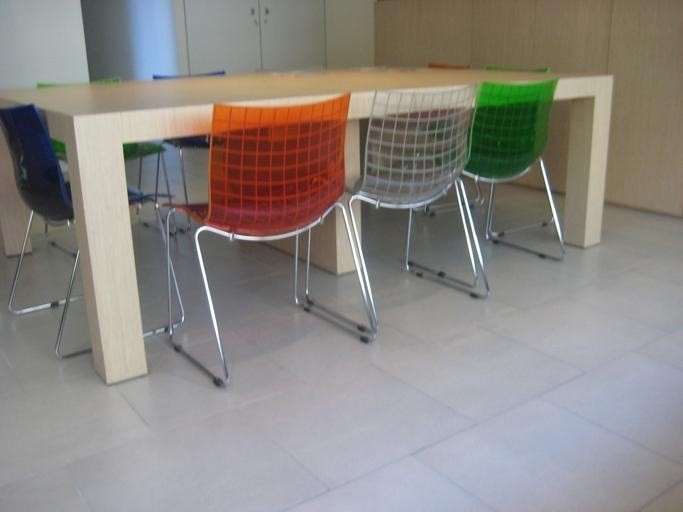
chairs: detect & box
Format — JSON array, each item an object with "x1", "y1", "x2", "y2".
[
  {"x1": 2, "y1": 104, "x2": 185, "y2": 359},
  {"x1": 304, "y1": 82, "x2": 477, "y2": 337},
  {"x1": 406, "y1": 77, "x2": 567, "y2": 289},
  {"x1": 166, "y1": 89, "x2": 377, "y2": 386}
]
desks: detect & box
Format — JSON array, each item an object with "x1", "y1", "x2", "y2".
[{"x1": 2, "y1": 63, "x2": 612, "y2": 389}]
